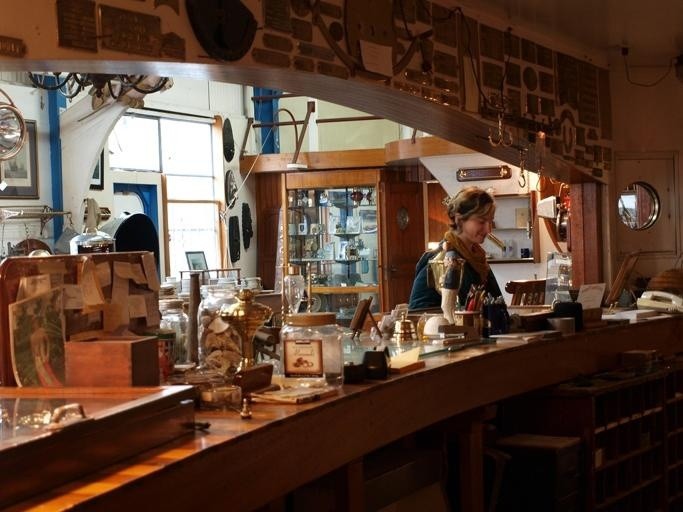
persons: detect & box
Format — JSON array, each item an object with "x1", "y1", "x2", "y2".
[{"x1": 408, "y1": 186, "x2": 511, "y2": 335}]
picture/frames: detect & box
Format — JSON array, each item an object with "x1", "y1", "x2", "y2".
[
  {"x1": 0, "y1": 119, "x2": 40, "y2": 200},
  {"x1": 185, "y1": 250, "x2": 210, "y2": 280},
  {"x1": 90, "y1": 146, "x2": 104, "y2": 191}
]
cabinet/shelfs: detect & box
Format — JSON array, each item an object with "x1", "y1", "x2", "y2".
[
  {"x1": 255, "y1": 165, "x2": 411, "y2": 323},
  {"x1": 480, "y1": 190, "x2": 540, "y2": 264},
  {"x1": 495, "y1": 356, "x2": 683, "y2": 511}
]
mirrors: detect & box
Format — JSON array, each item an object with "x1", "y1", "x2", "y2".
[{"x1": 618, "y1": 181, "x2": 660, "y2": 230}]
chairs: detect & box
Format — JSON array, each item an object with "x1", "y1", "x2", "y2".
[{"x1": 505, "y1": 277, "x2": 559, "y2": 306}]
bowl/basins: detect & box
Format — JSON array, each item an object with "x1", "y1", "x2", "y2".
[{"x1": 417, "y1": 309, "x2": 448, "y2": 342}]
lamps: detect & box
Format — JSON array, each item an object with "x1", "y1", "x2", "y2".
[{"x1": 0, "y1": 87, "x2": 26, "y2": 161}]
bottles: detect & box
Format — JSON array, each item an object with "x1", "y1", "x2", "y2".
[
  {"x1": 345, "y1": 239, "x2": 356, "y2": 261},
  {"x1": 280, "y1": 312, "x2": 344, "y2": 390},
  {"x1": 154, "y1": 286, "x2": 243, "y2": 385},
  {"x1": 500, "y1": 239, "x2": 516, "y2": 258}
]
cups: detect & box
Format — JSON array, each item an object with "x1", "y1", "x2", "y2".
[
  {"x1": 310, "y1": 224, "x2": 321, "y2": 235},
  {"x1": 553, "y1": 302, "x2": 584, "y2": 330},
  {"x1": 520, "y1": 247, "x2": 529, "y2": 258},
  {"x1": 452, "y1": 304, "x2": 510, "y2": 341}
]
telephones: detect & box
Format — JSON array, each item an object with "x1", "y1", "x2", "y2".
[{"x1": 636, "y1": 290, "x2": 683, "y2": 313}]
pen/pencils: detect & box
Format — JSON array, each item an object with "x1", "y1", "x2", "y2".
[
  {"x1": 464, "y1": 284, "x2": 503, "y2": 311},
  {"x1": 297, "y1": 394, "x2": 319, "y2": 404}
]
